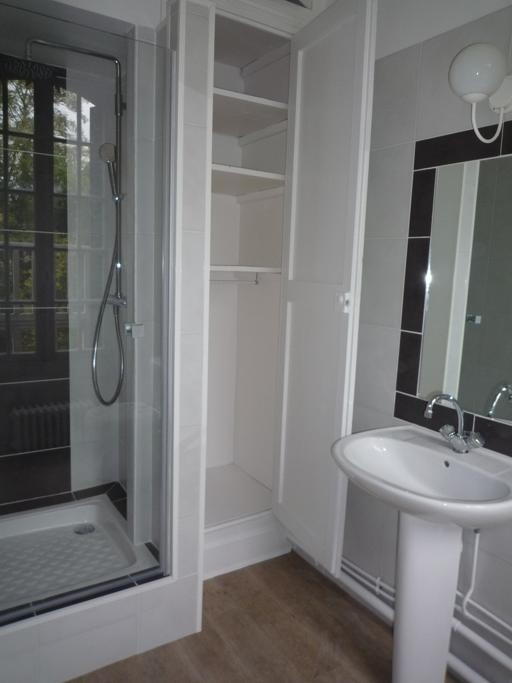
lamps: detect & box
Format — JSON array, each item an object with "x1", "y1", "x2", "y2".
[{"x1": 444, "y1": 40, "x2": 511, "y2": 144}]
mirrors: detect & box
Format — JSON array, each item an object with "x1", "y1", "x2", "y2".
[{"x1": 391, "y1": 118, "x2": 511, "y2": 456}]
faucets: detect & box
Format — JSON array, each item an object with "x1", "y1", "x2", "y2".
[
  {"x1": 487, "y1": 383, "x2": 512, "y2": 419},
  {"x1": 421, "y1": 393, "x2": 464, "y2": 440}
]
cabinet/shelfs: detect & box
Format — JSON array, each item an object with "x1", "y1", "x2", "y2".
[{"x1": 180, "y1": 1, "x2": 376, "y2": 585}]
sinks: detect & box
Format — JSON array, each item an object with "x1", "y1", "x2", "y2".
[{"x1": 331, "y1": 425, "x2": 512, "y2": 530}]
